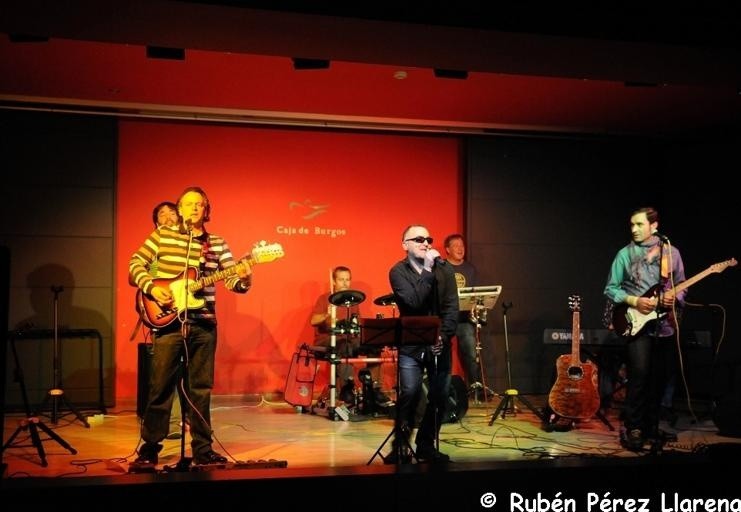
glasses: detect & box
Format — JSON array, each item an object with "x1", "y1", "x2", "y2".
[{"x1": 406, "y1": 236, "x2": 433, "y2": 244}]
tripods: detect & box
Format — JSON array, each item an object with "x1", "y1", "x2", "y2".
[
  {"x1": 0, "y1": 336, "x2": 76, "y2": 467},
  {"x1": 485, "y1": 311, "x2": 541, "y2": 423},
  {"x1": 35, "y1": 294, "x2": 91, "y2": 431}
]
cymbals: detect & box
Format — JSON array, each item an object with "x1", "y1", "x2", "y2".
[
  {"x1": 328, "y1": 290, "x2": 366, "y2": 307},
  {"x1": 374, "y1": 293, "x2": 396, "y2": 305}
]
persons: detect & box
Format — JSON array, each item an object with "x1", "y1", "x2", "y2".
[
  {"x1": 309, "y1": 266, "x2": 391, "y2": 402},
  {"x1": 150, "y1": 201, "x2": 184, "y2": 439},
  {"x1": 603, "y1": 206, "x2": 688, "y2": 452},
  {"x1": 436, "y1": 234, "x2": 495, "y2": 404},
  {"x1": 122, "y1": 187, "x2": 252, "y2": 465},
  {"x1": 382, "y1": 223, "x2": 449, "y2": 465}
]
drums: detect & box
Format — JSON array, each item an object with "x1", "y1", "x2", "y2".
[{"x1": 336, "y1": 312, "x2": 360, "y2": 335}]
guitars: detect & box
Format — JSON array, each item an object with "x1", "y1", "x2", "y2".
[
  {"x1": 549, "y1": 294, "x2": 600, "y2": 418},
  {"x1": 136, "y1": 239, "x2": 284, "y2": 329},
  {"x1": 611, "y1": 258, "x2": 738, "y2": 342}
]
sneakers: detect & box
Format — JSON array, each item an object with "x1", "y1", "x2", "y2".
[
  {"x1": 469, "y1": 387, "x2": 493, "y2": 401},
  {"x1": 339, "y1": 385, "x2": 355, "y2": 403},
  {"x1": 373, "y1": 388, "x2": 388, "y2": 403},
  {"x1": 416, "y1": 440, "x2": 449, "y2": 460},
  {"x1": 384, "y1": 441, "x2": 409, "y2": 464},
  {"x1": 167, "y1": 423, "x2": 183, "y2": 438},
  {"x1": 627, "y1": 422, "x2": 677, "y2": 452}
]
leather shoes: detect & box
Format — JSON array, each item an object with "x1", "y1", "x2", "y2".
[
  {"x1": 133, "y1": 456, "x2": 155, "y2": 469},
  {"x1": 193, "y1": 451, "x2": 227, "y2": 464}
]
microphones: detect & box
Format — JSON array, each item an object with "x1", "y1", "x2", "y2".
[
  {"x1": 426, "y1": 249, "x2": 449, "y2": 266},
  {"x1": 181, "y1": 217, "x2": 197, "y2": 229}
]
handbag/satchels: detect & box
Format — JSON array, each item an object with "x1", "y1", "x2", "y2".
[{"x1": 285, "y1": 344, "x2": 317, "y2": 407}]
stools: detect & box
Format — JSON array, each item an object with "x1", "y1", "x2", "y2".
[{"x1": 297, "y1": 343, "x2": 331, "y2": 409}]
